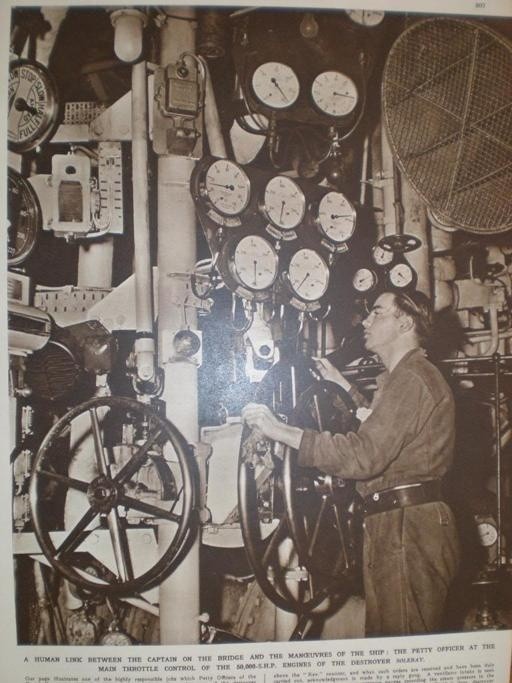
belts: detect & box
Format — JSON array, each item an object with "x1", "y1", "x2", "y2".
[{"x1": 360, "y1": 482, "x2": 440, "y2": 518}]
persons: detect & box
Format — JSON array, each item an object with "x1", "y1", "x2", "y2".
[{"x1": 241, "y1": 289, "x2": 458, "y2": 638}]
